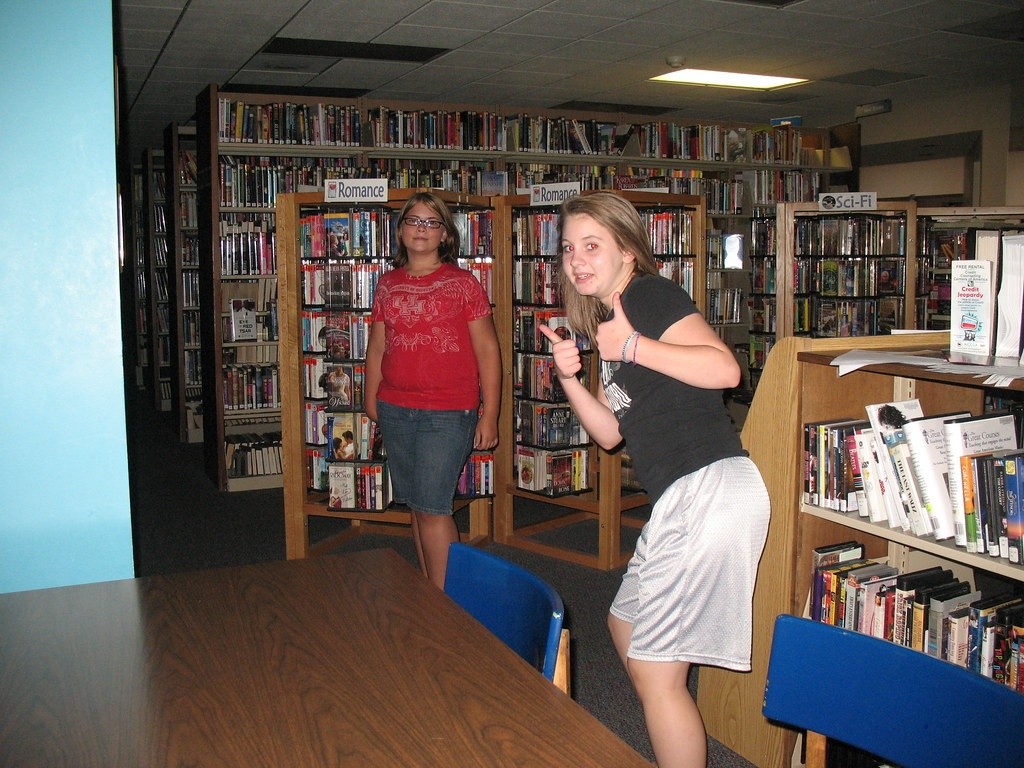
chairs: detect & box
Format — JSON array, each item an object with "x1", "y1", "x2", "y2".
[
  {"x1": 762, "y1": 614, "x2": 1024, "y2": 768},
  {"x1": 443, "y1": 542, "x2": 569, "y2": 700}
]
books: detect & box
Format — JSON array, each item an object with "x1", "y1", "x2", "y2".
[{"x1": 133, "y1": 99, "x2": 1024, "y2": 698}]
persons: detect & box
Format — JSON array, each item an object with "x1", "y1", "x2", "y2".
[
  {"x1": 365, "y1": 193, "x2": 502, "y2": 593},
  {"x1": 539, "y1": 193, "x2": 772, "y2": 768}
]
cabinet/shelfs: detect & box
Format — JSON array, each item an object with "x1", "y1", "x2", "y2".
[
  {"x1": 141, "y1": 148, "x2": 170, "y2": 411},
  {"x1": 126, "y1": 165, "x2": 147, "y2": 385},
  {"x1": 491, "y1": 188, "x2": 707, "y2": 571},
  {"x1": 164, "y1": 121, "x2": 203, "y2": 443},
  {"x1": 916, "y1": 207, "x2": 1024, "y2": 330},
  {"x1": 196, "y1": 84, "x2": 860, "y2": 493},
  {"x1": 795, "y1": 346, "x2": 1024, "y2": 768},
  {"x1": 774, "y1": 201, "x2": 916, "y2": 340},
  {"x1": 276, "y1": 192, "x2": 490, "y2": 560}
]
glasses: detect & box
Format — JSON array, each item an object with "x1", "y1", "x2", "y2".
[{"x1": 401, "y1": 218, "x2": 448, "y2": 229}]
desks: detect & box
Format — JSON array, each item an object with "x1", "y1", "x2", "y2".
[{"x1": 0, "y1": 548, "x2": 658, "y2": 768}]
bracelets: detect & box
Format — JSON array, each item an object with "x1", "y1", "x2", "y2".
[{"x1": 622, "y1": 331, "x2": 640, "y2": 366}]
